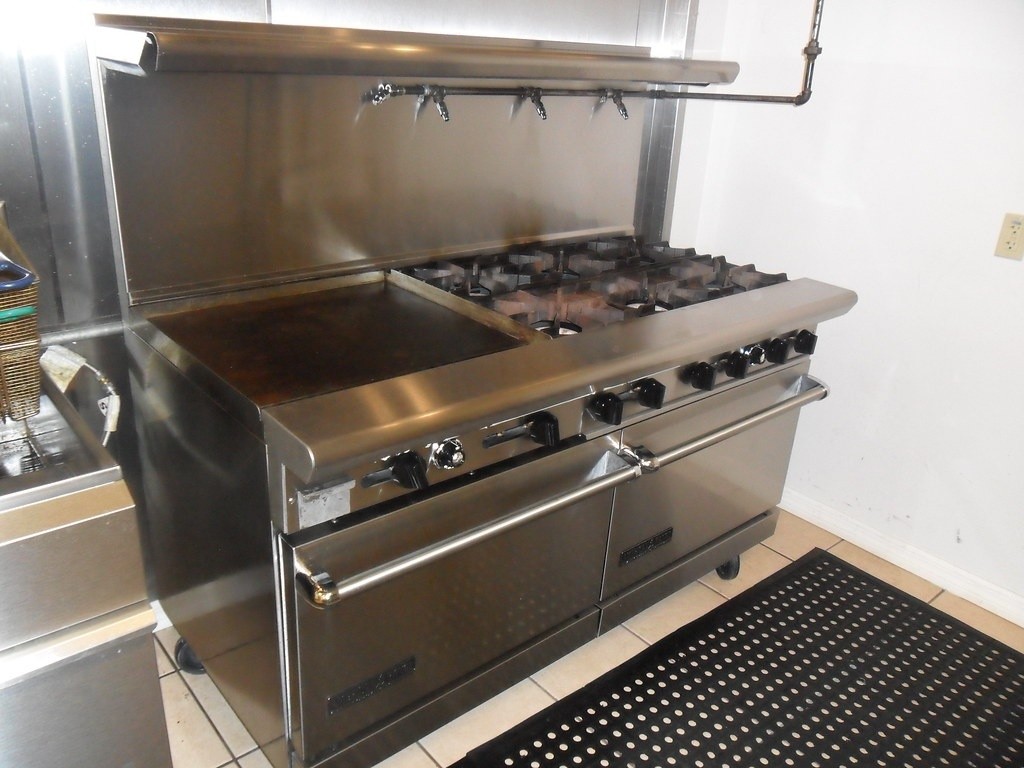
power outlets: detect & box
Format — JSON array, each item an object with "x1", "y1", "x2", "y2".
[{"x1": 994, "y1": 213, "x2": 1024, "y2": 261}]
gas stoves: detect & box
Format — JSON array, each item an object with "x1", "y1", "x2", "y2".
[{"x1": 390, "y1": 224, "x2": 791, "y2": 339}]
